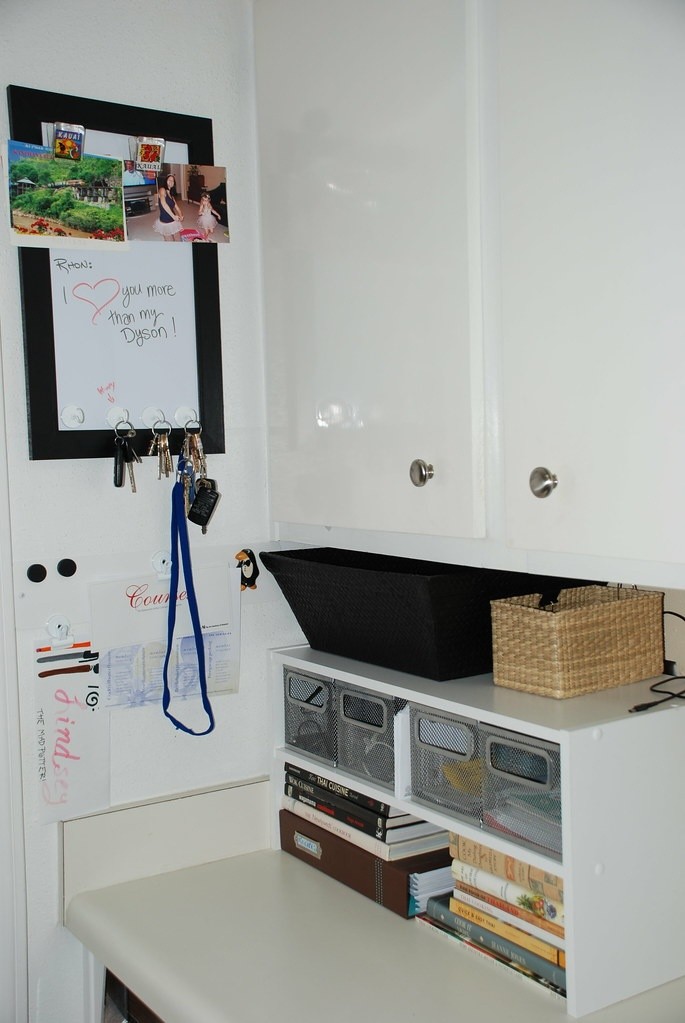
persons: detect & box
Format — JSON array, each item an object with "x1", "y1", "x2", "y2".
[
  {"x1": 195, "y1": 193, "x2": 221, "y2": 238},
  {"x1": 124, "y1": 161, "x2": 146, "y2": 186},
  {"x1": 152, "y1": 174, "x2": 184, "y2": 242}
]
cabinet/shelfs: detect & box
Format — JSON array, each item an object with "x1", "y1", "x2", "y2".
[
  {"x1": 255, "y1": 1, "x2": 684, "y2": 589},
  {"x1": 61, "y1": 645, "x2": 684, "y2": 1023}
]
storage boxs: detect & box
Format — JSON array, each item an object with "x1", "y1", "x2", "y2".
[
  {"x1": 490, "y1": 585, "x2": 664, "y2": 699},
  {"x1": 259, "y1": 547, "x2": 609, "y2": 683}
]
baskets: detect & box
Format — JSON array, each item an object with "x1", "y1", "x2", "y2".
[{"x1": 491, "y1": 584, "x2": 666, "y2": 700}]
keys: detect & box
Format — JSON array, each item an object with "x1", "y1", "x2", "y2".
[
  {"x1": 182, "y1": 435, "x2": 219, "y2": 534},
  {"x1": 114, "y1": 440, "x2": 140, "y2": 493},
  {"x1": 148, "y1": 435, "x2": 176, "y2": 480}
]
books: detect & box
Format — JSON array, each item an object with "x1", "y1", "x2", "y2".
[
  {"x1": 416, "y1": 832, "x2": 566, "y2": 997},
  {"x1": 280, "y1": 762, "x2": 457, "y2": 920}
]
